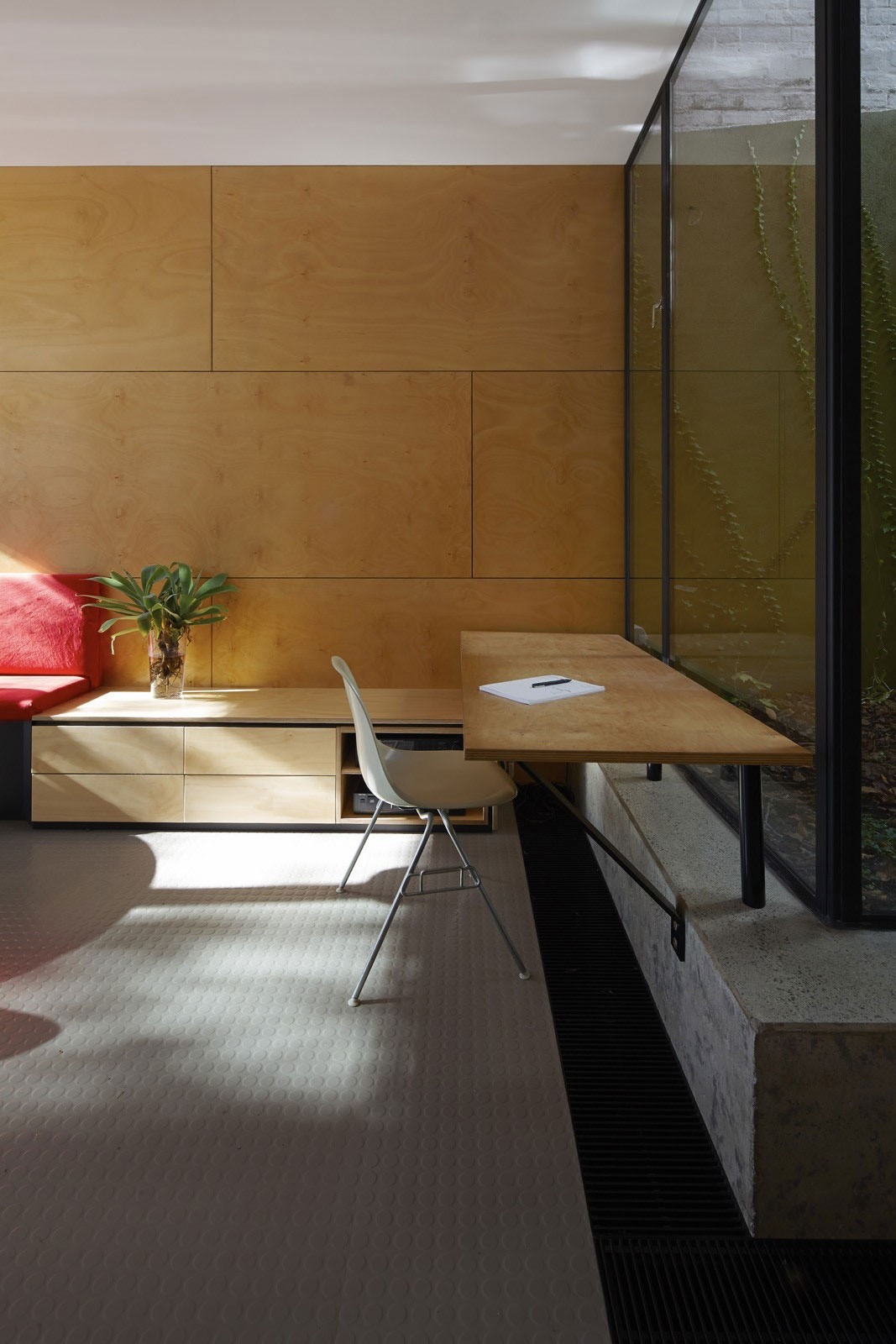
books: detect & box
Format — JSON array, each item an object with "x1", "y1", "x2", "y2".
[{"x1": 480, "y1": 675, "x2": 607, "y2": 705}]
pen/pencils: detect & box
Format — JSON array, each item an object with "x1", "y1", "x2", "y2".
[{"x1": 532, "y1": 679, "x2": 572, "y2": 686}]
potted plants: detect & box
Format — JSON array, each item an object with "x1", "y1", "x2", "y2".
[{"x1": 74, "y1": 557, "x2": 245, "y2": 699}]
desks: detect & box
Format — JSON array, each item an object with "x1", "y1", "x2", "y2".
[{"x1": 460, "y1": 631, "x2": 816, "y2": 910}]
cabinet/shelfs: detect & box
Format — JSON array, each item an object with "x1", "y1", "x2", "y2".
[{"x1": 22, "y1": 685, "x2": 505, "y2": 836}]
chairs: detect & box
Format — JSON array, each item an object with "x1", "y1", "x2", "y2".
[
  {"x1": 0, "y1": 572, "x2": 107, "y2": 823},
  {"x1": 332, "y1": 654, "x2": 532, "y2": 1006}
]
